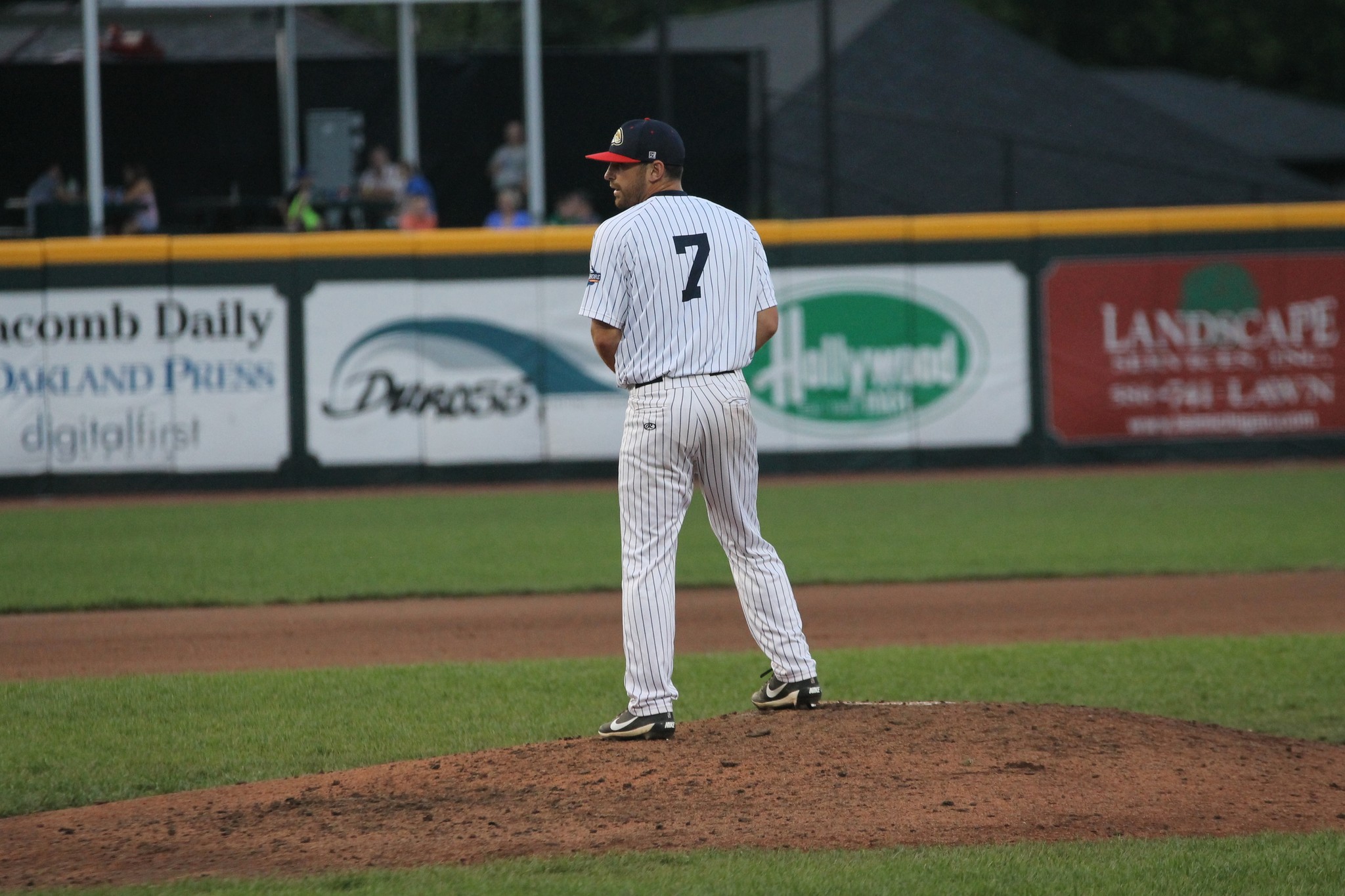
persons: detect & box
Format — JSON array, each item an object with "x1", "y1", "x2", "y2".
[
  {"x1": 286, "y1": 147, "x2": 437, "y2": 231},
  {"x1": 29, "y1": 164, "x2": 158, "y2": 236},
  {"x1": 482, "y1": 124, "x2": 534, "y2": 227},
  {"x1": 580, "y1": 118, "x2": 822, "y2": 738},
  {"x1": 552, "y1": 195, "x2": 600, "y2": 226}
]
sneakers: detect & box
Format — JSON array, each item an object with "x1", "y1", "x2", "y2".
[
  {"x1": 598, "y1": 700, "x2": 675, "y2": 741},
  {"x1": 752, "y1": 664, "x2": 820, "y2": 710}
]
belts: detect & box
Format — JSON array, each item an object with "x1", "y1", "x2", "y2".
[{"x1": 635, "y1": 367, "x2": 736, "y2": 389}]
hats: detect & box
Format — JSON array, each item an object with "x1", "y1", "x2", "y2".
[{"x1": 585, "y1": 118, "x2": 687, "y2": 168}]
